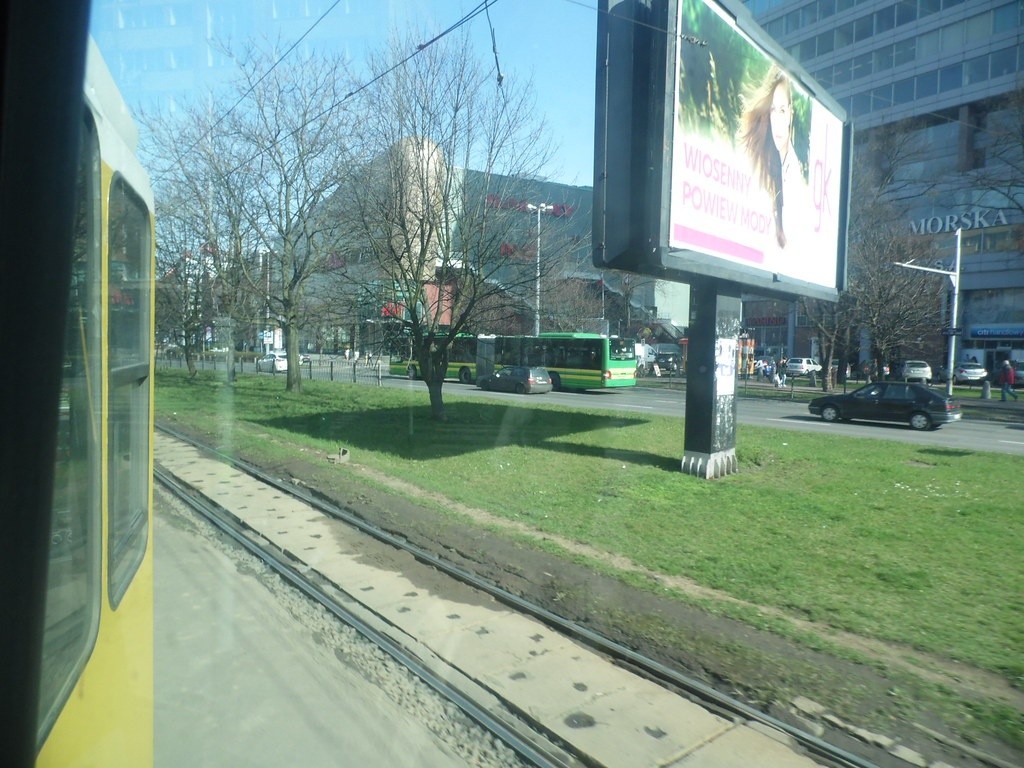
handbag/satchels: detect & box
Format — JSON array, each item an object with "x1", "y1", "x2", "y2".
[{"x1": 773, "y1": 373, "x2": 781, "y2": 385}]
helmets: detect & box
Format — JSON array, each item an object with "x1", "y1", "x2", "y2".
[{"x1": 1002, "y1": 360, "x2": 1009, "y2": 366}]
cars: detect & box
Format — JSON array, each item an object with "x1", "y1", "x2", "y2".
[
  {"x1": 1013, "y1": 361, "x2": 1024, "y2": 386},
  {"x1": 856, "y1": 359, "x2": 890, "y2": 377},
  {"x1": 257, "y1": 353, "x2": 288, "y2": 373},
  {"x1": 786, "y1": 358, "x2": 822, "y2": 375},
  {"x1": 654, "y1": 352, "x2": 684, "y2": 371},
  {"x1": 831, "y1": 359, "x2": 851, "y2": 379},
  {"x1": 937, "y1": 363, "x2": 988, "y2": 386},
  {"x1": 902, "y1": 360, "x2": 932, "y2": 383},
  {"x1": 809, "y1": 382, "x2": 962, "y2": 431},
  {"x1": 481, "y1": 366, "x2": 553, "y2": 394}
]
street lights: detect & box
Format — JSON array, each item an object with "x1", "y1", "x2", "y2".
[
  {"x1": 259, "y1": 249, "x2": 278, "y2": 355},
  {"x1": 526, "y1": 203, "x2": 554, "y2": 336}
]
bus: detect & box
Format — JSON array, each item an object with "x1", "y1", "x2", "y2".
[
  {"x1": 475, "y1": 332, "x2": 637, "y2": 392},
  {"x1": 388, "y1": 333, "x2": 477, "y2": 384}
]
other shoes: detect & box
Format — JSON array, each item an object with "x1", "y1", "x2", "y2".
[
  {"x1": 998, "y1": 400, "x2": 1006, "y2": 401},
  {"x1": 1015, "y1": 396, "x2": 1019, "y2": 401}
]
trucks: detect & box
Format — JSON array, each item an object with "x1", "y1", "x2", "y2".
[{"x1": 616, "y1": 343, "x2": 657, "y2": 363}]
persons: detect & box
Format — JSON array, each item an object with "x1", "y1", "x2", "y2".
[
  {"x1": 746, "y1": 354, "x2": 788, "y2": 387},
  {"x1": 741, "y1": 66, "x2": 825, "y2": 265},
  {"x1": 1000, "y1": 360, "x2": 1018, "y2": 403},
  {"x1": 367, "y1": 350, "x2": 374, "y2": 366},
  {"x1": 344, "y1": 348, "x2": 360, "y2": 365}
]
